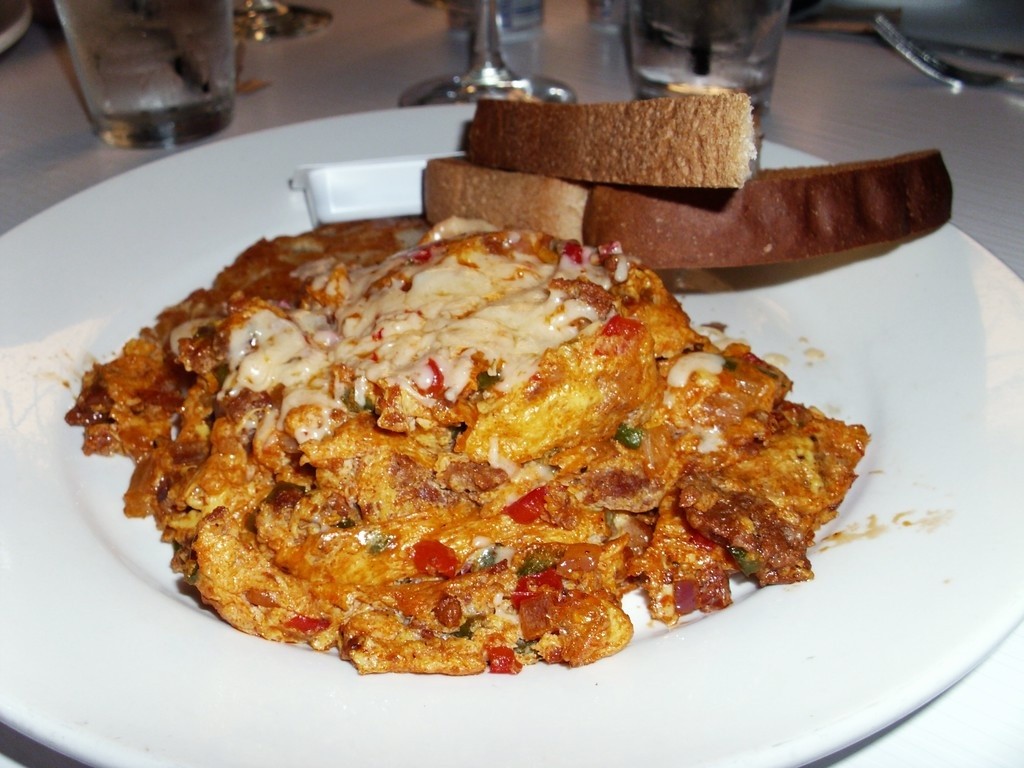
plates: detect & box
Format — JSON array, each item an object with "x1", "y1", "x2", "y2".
[
  {"x1": 0, "y1": 103, "x2": 1023, "y2": 768},
  {"x1": 0, "y1": 0, "x2": 32, "y2": 54}
]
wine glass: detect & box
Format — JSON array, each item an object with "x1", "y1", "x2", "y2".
[
  {"x1": 398, "y1": 1, "x2": 578, "y2": 107},
  {"x1": 232, "y1": 0, "x2": 333, "y2": 43}
]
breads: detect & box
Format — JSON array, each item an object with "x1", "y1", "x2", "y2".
[
  {"x1": 465, "y1": 91, "x2": 762, "y2": 190},
  {"x1": 423, "y1": 148, "x2": 953, "y2": 269}
]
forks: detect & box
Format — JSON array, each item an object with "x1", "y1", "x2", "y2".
[{"x1": 870, "y1": 11, "x2": 1024, "y2": 94}]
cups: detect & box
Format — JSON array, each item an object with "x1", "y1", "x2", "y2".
[
  {"x1": 56, "y1": 0, "x2": 235, "y2": 150},
  {"x1": 623, "y1": 0, "x2": 789, "y2": 141}
]
